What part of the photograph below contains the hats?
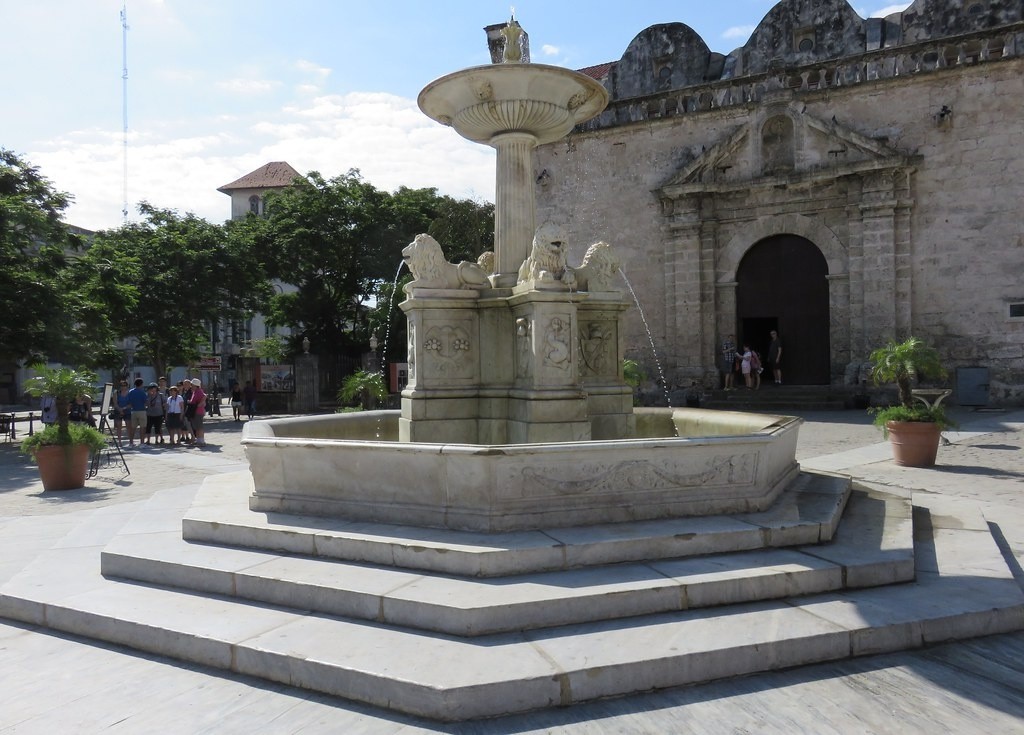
[190,378,201,387]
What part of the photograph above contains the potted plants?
[18,362,110,489]
[863,336,950,467]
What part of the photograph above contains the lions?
[516,223,575,284]
[402,233,493,294]
[574,241,619,292]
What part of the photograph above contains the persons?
[181,378,195,446]
[165,385,184,447]
[767,330,784,386]
[721,335,738,391]
[128,378,152,449]
[39,387,60,429]
[143,382,168,448]
[738,343,753,392]
[68,390,97,431]
[175,381,190,442]
[155,376,175,447]
[749,349,762,390]
[228,382,244,422]
[112,380,135,450]
[240,381,257,421]
[186,377,207,447]
